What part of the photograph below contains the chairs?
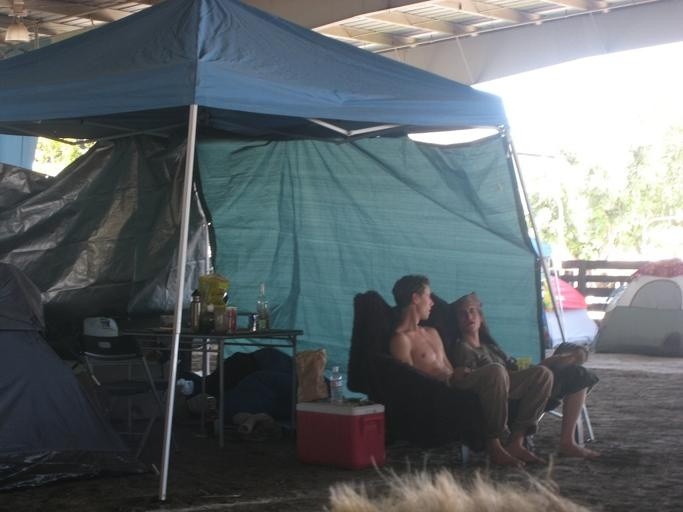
[51,307,175,465]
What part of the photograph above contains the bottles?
[255,282,268,332]
[189,295,201,328]
[463,356,477,376]
[329,366,342,404]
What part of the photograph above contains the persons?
[389,276,554,466]
[451,293,603,460]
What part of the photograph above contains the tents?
[0,262,150,492]
[593,259,683,357]
[540,276,599,349]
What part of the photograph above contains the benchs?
[334,282,599,483]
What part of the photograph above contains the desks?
[110,325,306,467]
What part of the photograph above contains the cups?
[159,313,174,329]
[516,357,532,369]
[248,314,257,332]
[199,312,215,334]
[224,307,239,332]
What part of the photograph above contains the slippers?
[481,452,528,471]
[516,451,548,465]
[559,445,601,460]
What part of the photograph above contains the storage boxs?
[291,397,389,473]
